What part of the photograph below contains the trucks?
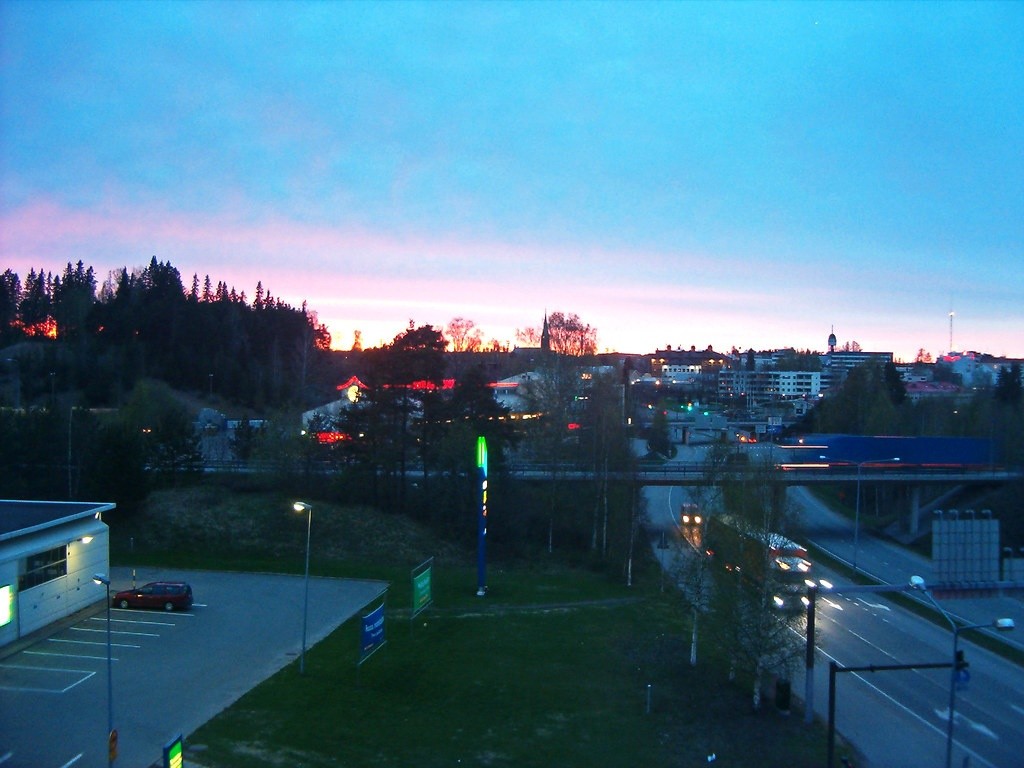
[706,511,809,614]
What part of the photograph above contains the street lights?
[820,454,901,576]
[294,501,311,676]
[910,575,1015,768]
[93,573,115,768]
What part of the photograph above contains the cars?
[682,503,701,525]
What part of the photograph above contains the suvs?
[112,581,193,612]
